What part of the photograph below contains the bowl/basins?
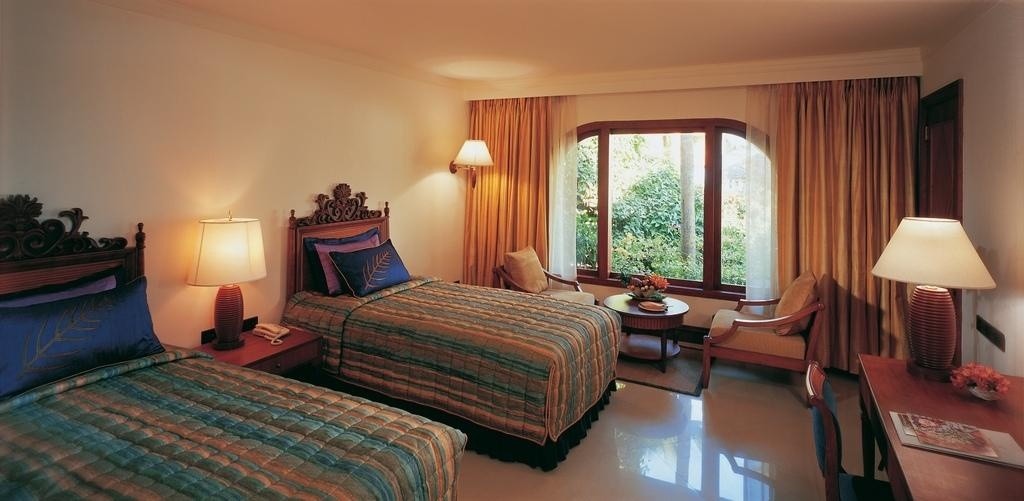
[626,292,667,301]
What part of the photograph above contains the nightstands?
[191,323,324,387]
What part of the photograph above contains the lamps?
[449,139,495,189]
[871,216,996,381]
[185,209,268,352]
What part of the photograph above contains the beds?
[279,184,623,472]
[0,193,468,501]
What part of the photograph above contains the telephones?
[253,323,290,340]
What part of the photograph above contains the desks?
[603,293,690,374]
[856,353,1024,501]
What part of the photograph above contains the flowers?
[948,362,1010,401]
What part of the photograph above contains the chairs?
[804,359,897,501]
[492,263,600,307]
[702,296,824,409]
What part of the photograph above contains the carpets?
[615,345,716,397]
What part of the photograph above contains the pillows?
[774,268,819,336]
[503,245,549,295]
[303,227,413,299]
[0,264,165,400]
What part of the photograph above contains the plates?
[638,301,668,312]
